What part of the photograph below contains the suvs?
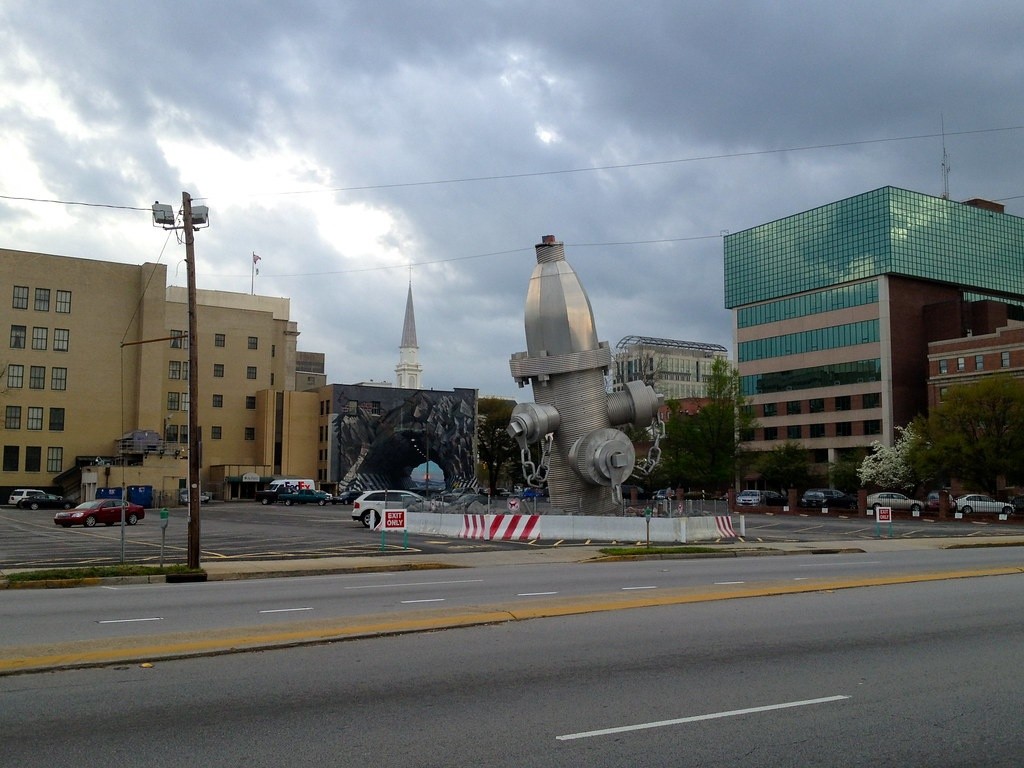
[351,490,451,531]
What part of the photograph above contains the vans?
[802,489,848,508]
[264,479,316,505]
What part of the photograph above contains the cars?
[8,488,48,506]
[759,491,784,505]
[428,486,549,502]
[176,487,189,506]
[17,494,78,510]
[332,491,363,505]
[621,485,644,498]
[651,489,675,500]
[953,493,1014,515]
[313,490,333,501]
[737,489,762,507]
[51,498,145,527]
[861,492,925,511]
[926,490,956,511]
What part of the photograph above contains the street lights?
[146,188,211,586]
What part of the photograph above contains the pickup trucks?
[254,487,299,506]
[277,488,330,507]
